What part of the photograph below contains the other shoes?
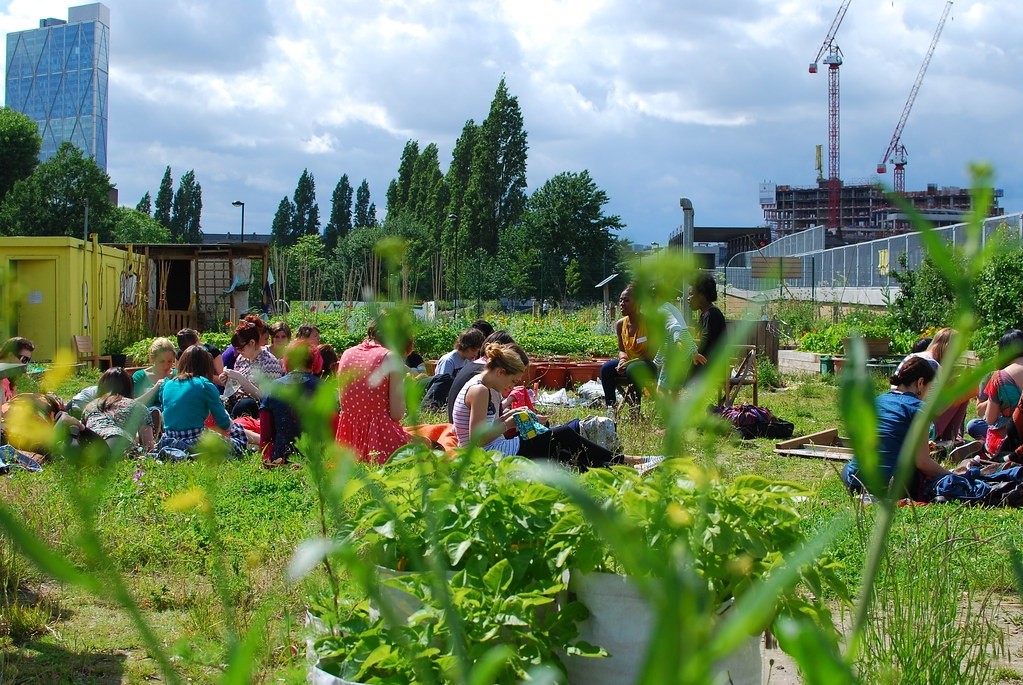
[638,455,673,478]
[607,406,614,417]
[139,409,160,445]
[949,441,982,465]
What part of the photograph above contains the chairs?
[615,344,758,424]
[73,335,113,371]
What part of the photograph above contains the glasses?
[235,342,248,351]
[14,353,31,364]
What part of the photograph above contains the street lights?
[447,213,459,320]
[231,200,245,242]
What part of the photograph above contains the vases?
[519,355,613,388]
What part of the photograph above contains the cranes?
[876,0,953,198]
[808,0,852,233]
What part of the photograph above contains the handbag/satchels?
[513,411,548,441]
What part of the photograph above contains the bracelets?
[502,422,508,431]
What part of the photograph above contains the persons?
[453,343,665,472]
[842,328,1023,505]
[66,312,445,466]
[0,337,36,476]
[434,320,542,423]
[600,273,728,425]
